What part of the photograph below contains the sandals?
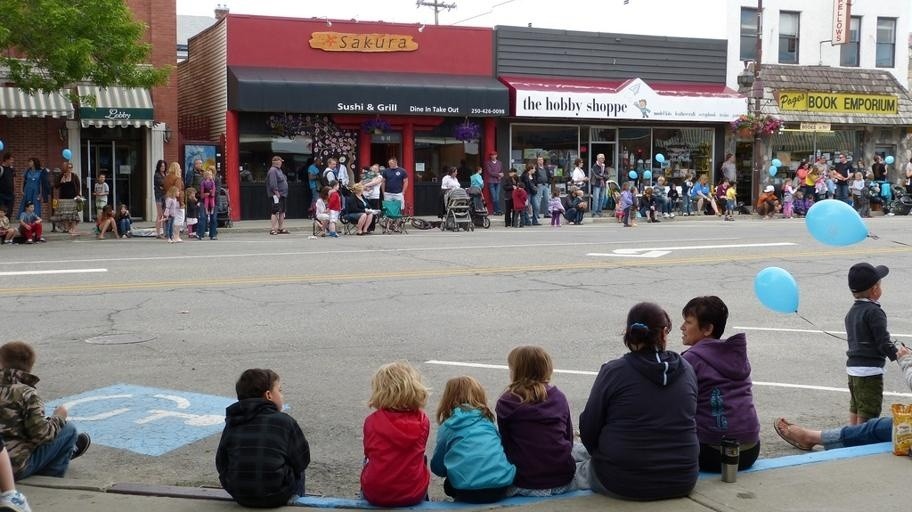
[277,229,290,233]
[270,229,277,235]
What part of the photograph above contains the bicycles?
[378,206,431,229]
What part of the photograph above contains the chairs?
[341,196,359,236]
[380,200,409,235]
[311,206,330,237]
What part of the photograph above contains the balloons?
[0,140,4,152]
[655,153,665,163]
[643,169,653,181]
[771,158,783,168]
[805,199,868,247]
[769,166,778,178]
[885,155,896,166]
[629,170,638,180]
[62,148,72,161]
[754,266,800,315]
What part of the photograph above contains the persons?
[572,158,589,193]
[215,368,311,510]
[18,201,47,244]
[315,187,332,235]
[0,342,90,483]
[589,154,608,218]
[93,173,109,218]
[640,188,661,222]
[185,160,204,232]
[360,167,380,197]
[667,183,683,216]
[185,188,200,238]
[757,185,779,219]
[805,193,815,211]
[782,178,798,219]
[846,262,891,425]
[421,167,435,182]
[154,160,168,239]
[521,163,542,226]
[878,155,885,164]
[197,166,218,240]
[831,153,854,204]
[0,152,15,221]
[1,435,33,512]
[0,205,19,245]
[652,176,675,218]
[164,186,180,243]
[772,343,912,453]
[162,162,184,243]
[869,183,884,202]
[382,156,409,214]
[905,155,912,194]
[629,186,640,227]
[690,174,721,217]
[495,347,577,495]
[96,205,120,240]
[266,156,291,236]
[726,180,737,221]
[239,163,257,184]
[574,191,587,226]
[359,164,383,232]
[682,173,695,217]
[848,171,864,209]
[615,196,624,223]
[202,170,216,215]
[512,183,529,229]
[535,155,554,219]
[323,180,342,237]
[457,159,472,177]
[503,167,526,228]
[549,187,566,227]
[859,180,875,218]
[620,182,634,227]
[335,158,349,189]
[872,156,889,181]
[721,153,736,183]
[679,296,761,472]
[360,362,429,507]
[486,152,505,216]
[430,377,516,503]
[814,155,828,175]
[307,157,322,203]
[803,165,824,205]
[114,204,134,239]
[437,166,461,219]
[346,183,373,236]
[578,303,698,501]
[851,159,868,178]
[865,173,895,216]
[322,158,337,190]
[562,185,587,225]
[794,162,809,197]
[815,166,828,195]
[470,167,487,210]
[717,177,732,215]
[16,157,48,220]
[55,163,81,234]
[824,171,839,200]
[793,191,806,215]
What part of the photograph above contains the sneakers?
[0,490,29,512]
[71,433,91,459]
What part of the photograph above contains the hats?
[490,151,498,155]
[764,185,775,192]
[272,156,284,163]
[849,262,890,292]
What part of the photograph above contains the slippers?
[774,417,811,450]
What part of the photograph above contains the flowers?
[760,118,783,134]
[360,119,392,133]
[730,116,762,133]
[452,123,480,143]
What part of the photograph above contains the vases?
[738,128,754,137]
[372,128,384,135]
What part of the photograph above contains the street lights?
[737,59,761,213]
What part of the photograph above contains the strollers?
[466,187,490,228]
[216,187,231,228]
[439,189,474,232]
